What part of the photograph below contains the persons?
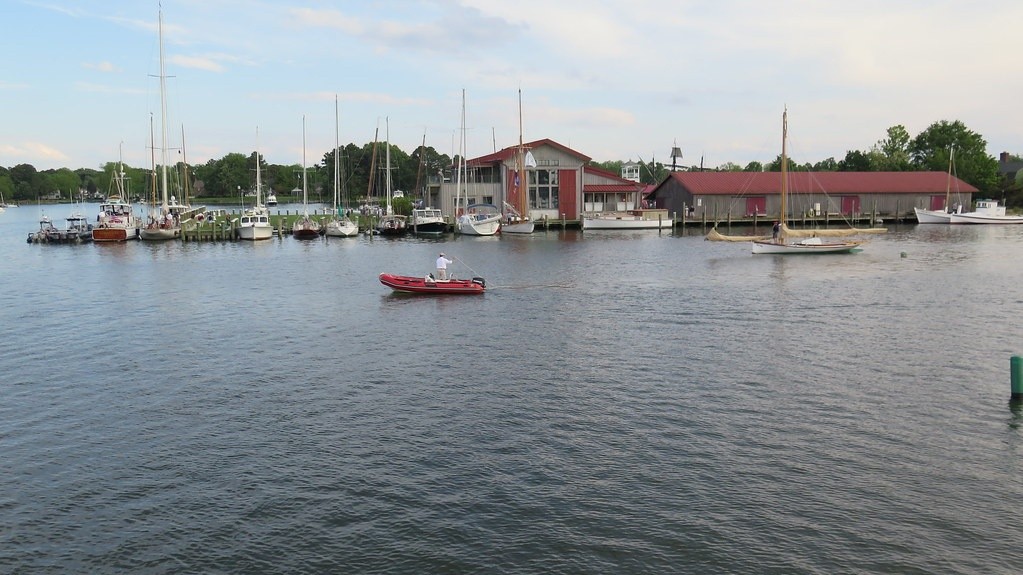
[191,212,195,221]
[117,207,123,214]
[166,212,172,219]
[773,222,779,241]
[685,205,689,219]
[689,204,695,219]
[346,210,350,217]
[436,253,452,280]
[226,213,231,225]
[207,213,212,228]
[198,211,204,228]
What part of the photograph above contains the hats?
[440,253,445,256]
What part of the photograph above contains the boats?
[379,272,488,295]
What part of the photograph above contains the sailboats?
[914,143,1023,225]
[27,1,273,242]
[291,113,325,238]
[703,101,867,254]
[0,191,20,209]
[267,187,278,206]
[361,86,535,237]
[326,94,360,237]
